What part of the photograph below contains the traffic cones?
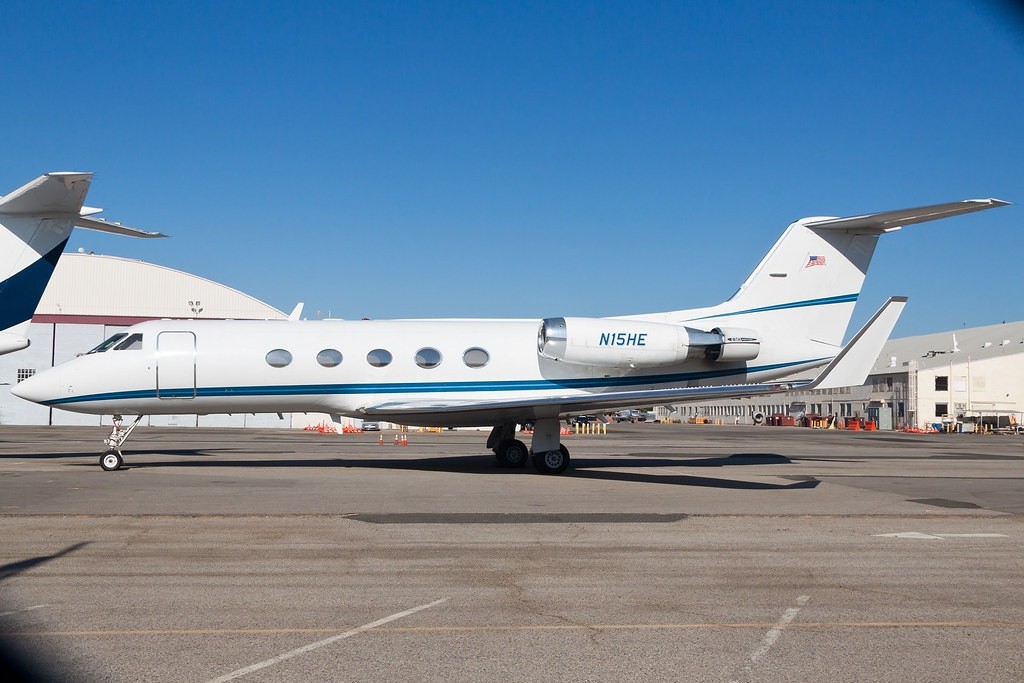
[401,434,405,447]
[379,432,383,446]
[393,435,399,445]
[404,435,408,447]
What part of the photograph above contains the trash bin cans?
[932,424,942,430]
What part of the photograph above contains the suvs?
[614,410,649,423]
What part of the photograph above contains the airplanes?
[0,172,1014,476]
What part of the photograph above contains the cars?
[571,416,590,427]
[361,421,380,430]
